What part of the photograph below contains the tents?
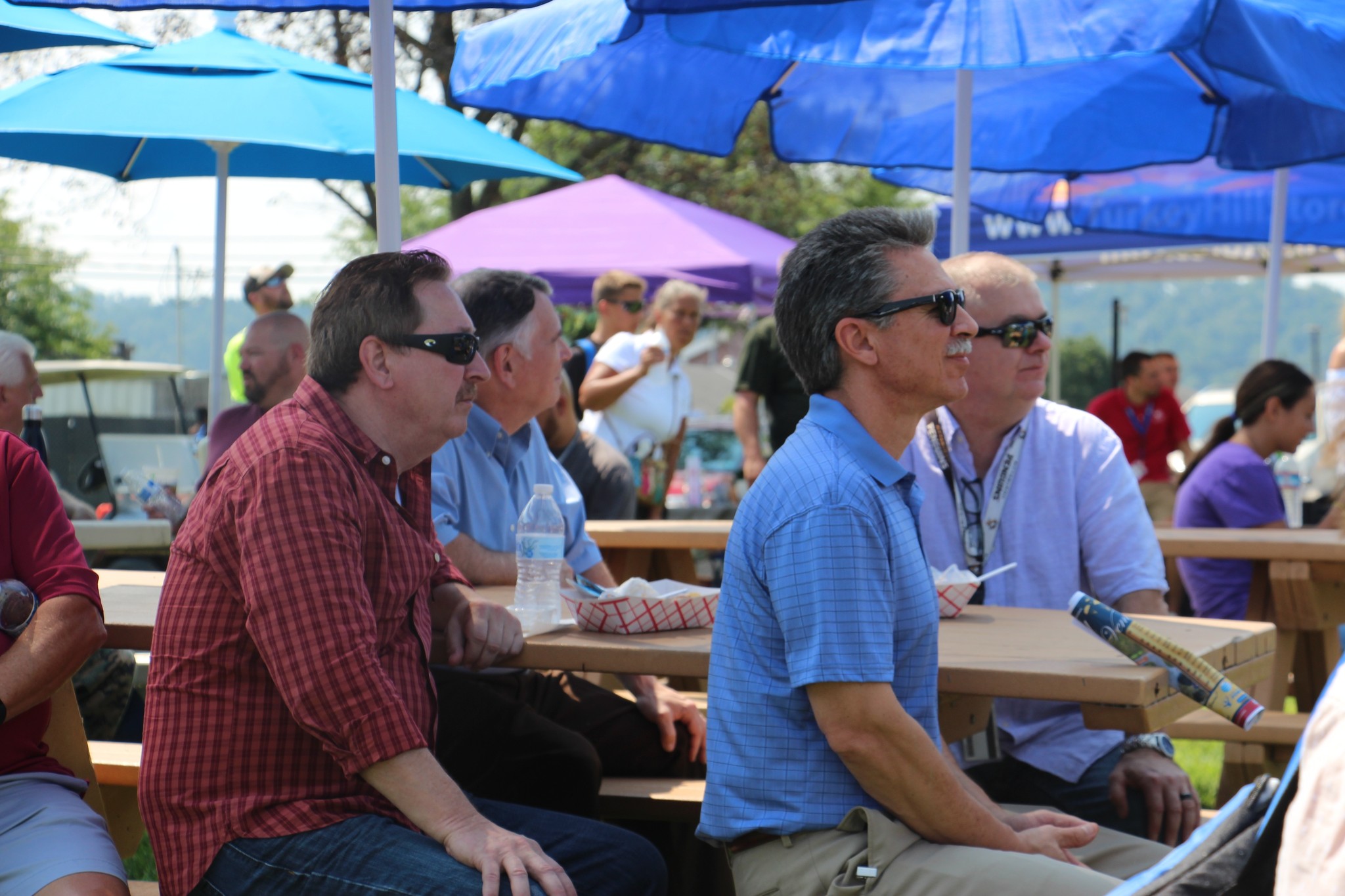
[401,171,806,308]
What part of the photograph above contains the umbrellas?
[0,0,1345,460]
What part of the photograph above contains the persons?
[0,249,1345,895]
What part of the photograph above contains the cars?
[1169,388,1237,472]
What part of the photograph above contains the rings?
[1180,793,1193,800]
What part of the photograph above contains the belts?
[724,828,796,856]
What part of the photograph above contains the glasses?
[865,289,965,326]
[607,300,642,313]
[390,332,480,365]
[262,275,284,288]
[961,477,984,559]
[973,314,1054,349]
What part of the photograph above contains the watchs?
[1122,731,1175,759]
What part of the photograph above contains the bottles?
[1273,451,1303,529]
[513,484,565,632]
[121,468,188,525]
[114,475,130,514]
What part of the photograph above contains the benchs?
[86,691,1309,803]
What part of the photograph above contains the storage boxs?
[561,578,720,634]
[936,570,980,618]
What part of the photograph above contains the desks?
[91,616,1345,810]
[491,520,1345,711]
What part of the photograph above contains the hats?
[244,264,293,297]
